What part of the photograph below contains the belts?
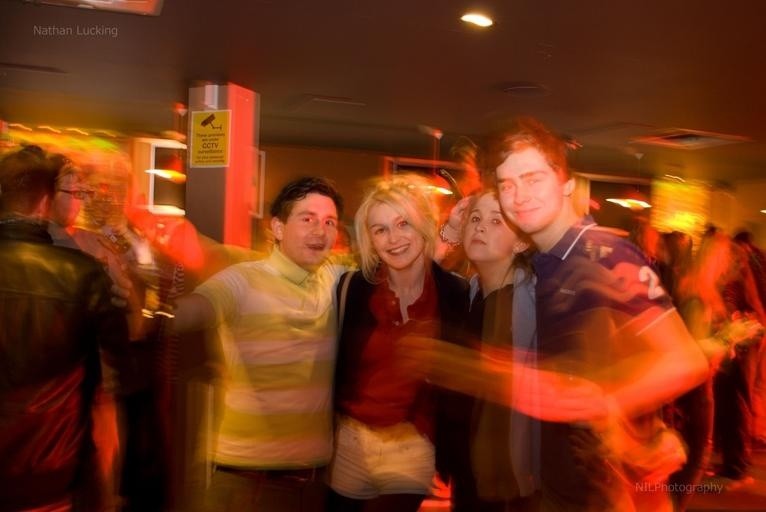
[213,463,328,484]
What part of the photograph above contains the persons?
[0,115,766,511]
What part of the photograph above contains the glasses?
[55,185,96,201]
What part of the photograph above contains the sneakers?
[696,460,753,493]
